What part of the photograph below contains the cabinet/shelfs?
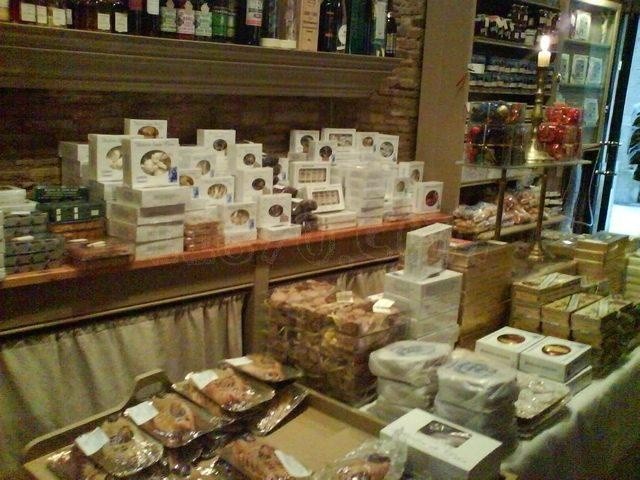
[415,0,567,242]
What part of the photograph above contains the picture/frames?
[560,9,603,124]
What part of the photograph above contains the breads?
[262,280,406,407]
[454,190,548,236]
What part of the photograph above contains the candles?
[537,34,552,67]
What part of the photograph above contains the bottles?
[384,8,397,57]
[475,4,558,51]
[470,54,555,89]
[1,0,298,46]
[319,0,339,53]
[335,0,347,52]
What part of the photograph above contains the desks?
[500,347,639,479]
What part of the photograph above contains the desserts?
[45,353,386,480]
